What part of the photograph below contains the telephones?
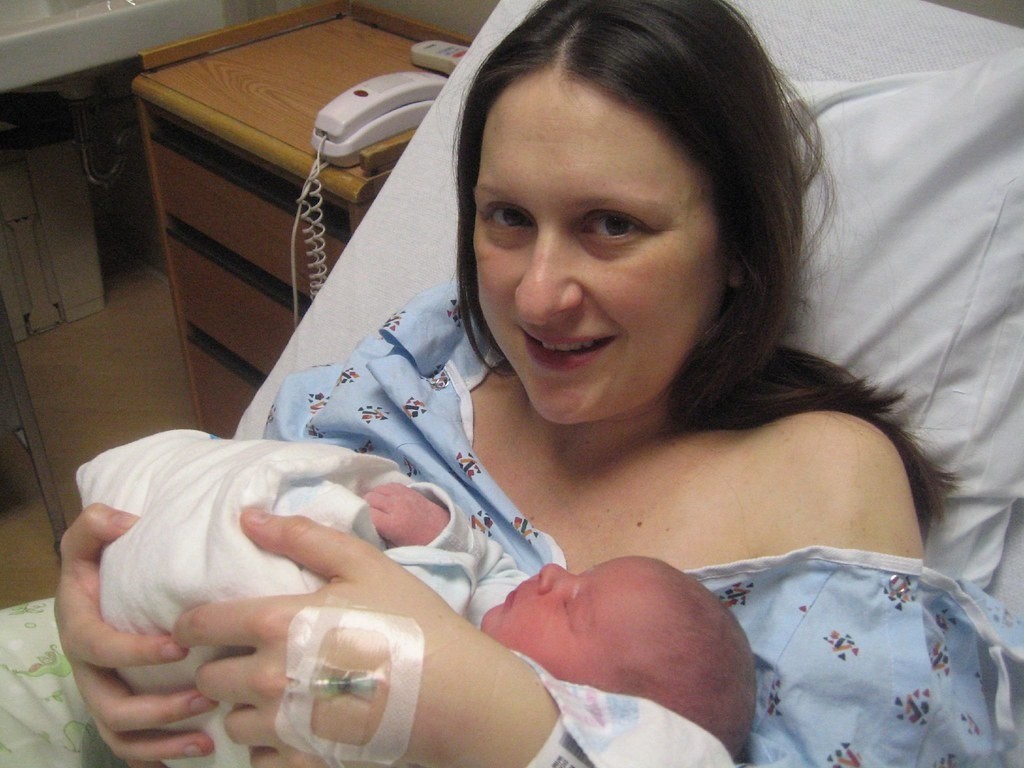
[307,63,452,170]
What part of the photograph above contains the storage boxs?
[0,91,106,345]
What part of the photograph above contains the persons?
[55,1,1023,767]
[76,428,757,768]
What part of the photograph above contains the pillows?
[779,43,1024,504]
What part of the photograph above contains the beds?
[0,0,1024,768]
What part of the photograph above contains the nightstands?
[135,0,478,440]
